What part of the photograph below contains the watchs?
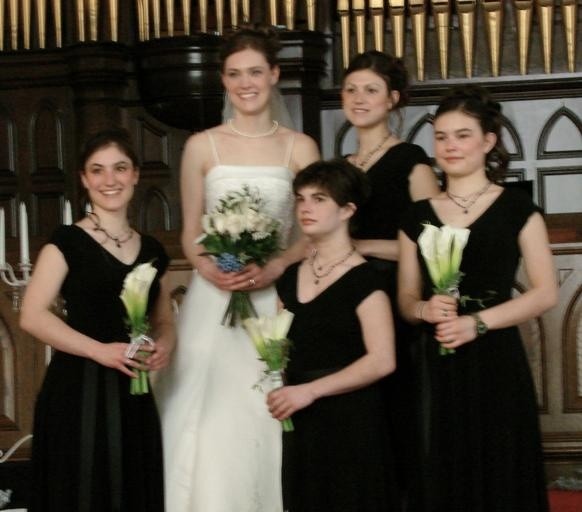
[469,312,488,336]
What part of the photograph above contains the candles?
[18,201,32,264]
[83,200,92,219]
[1,207,6,268]
[62,199,73,228]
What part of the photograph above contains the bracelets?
[416,301,425,320]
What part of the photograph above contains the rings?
[249,279,255,287]
[441,309,448,316]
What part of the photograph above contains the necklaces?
[82,211,134,248]
[449,191,477,203]
[311,246,355,285]
[314,257,340,271]
[446,181,495,215]
[225,115,281,140]
[352,134,394,170]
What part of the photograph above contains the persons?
[146,30,321,511]
[261,159,410,511]
[17,124,177,511]
[326,48,441,509]
[397,84,563,512]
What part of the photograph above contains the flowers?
[193,182,288,327]
[118,256,160,396]
[414,219,501,357]
[239,305,299,432]
